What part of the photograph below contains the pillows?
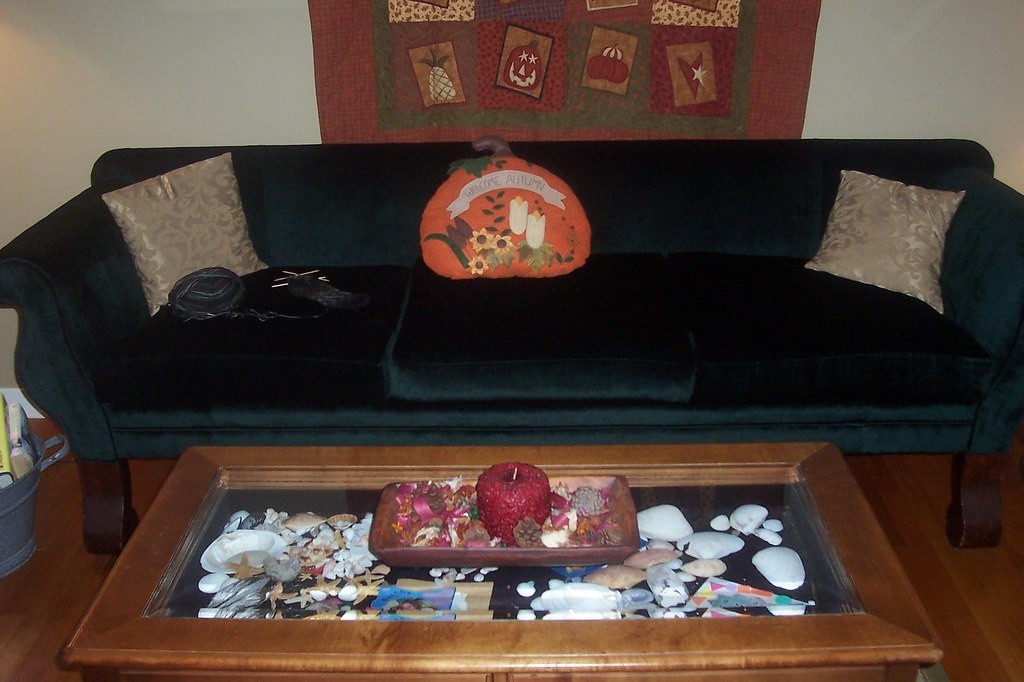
[806,168,967,315]
[412,138,591,279]
[100,150,268,317]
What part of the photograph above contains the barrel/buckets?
[0,430,48,580]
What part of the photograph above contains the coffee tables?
[56,441,945,682]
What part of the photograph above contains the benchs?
[0,139,1024,550]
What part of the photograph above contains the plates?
[368,473,641,568]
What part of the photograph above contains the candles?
[474,462,552,544]
